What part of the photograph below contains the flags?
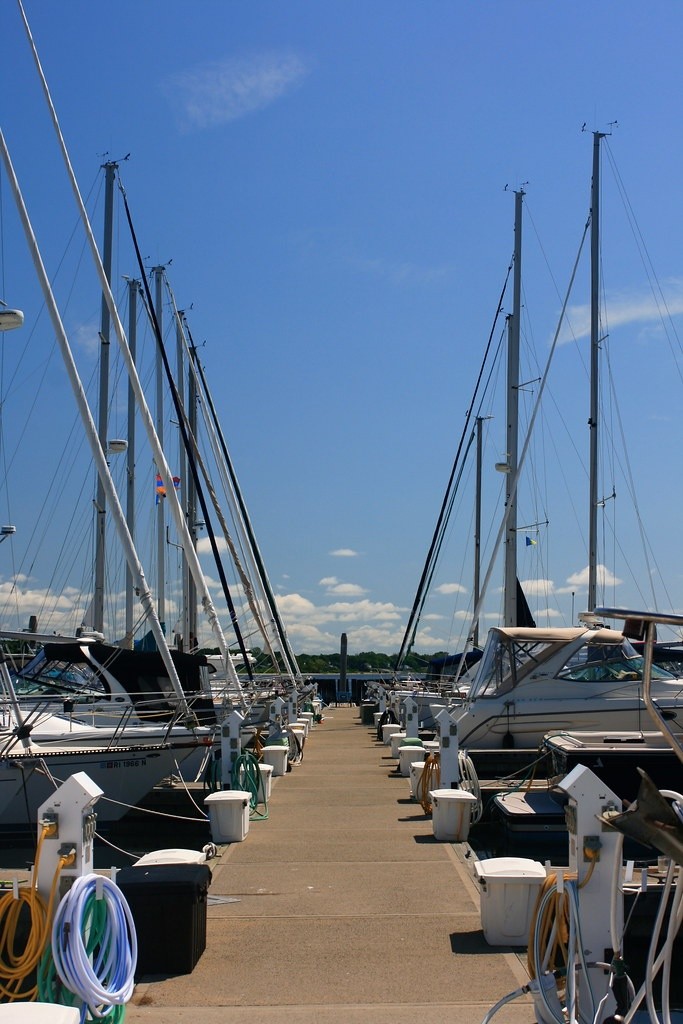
[526,536,537,547]
[155,475,179,506]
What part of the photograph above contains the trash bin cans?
[398,746,426,777]
[374,712,385,728]
[474,856,546,946]
[282,730,304,749]
[260,745,289,775]
[299,712,314,727]
[204,790,251,843]
[410,762,437,800]
[285,723,308,738]
[297,718,310,729]
[382,723,402,745]
[429,788,476,842]
[239,764,274,803]
[390,733,407,758]
[134,849,208,866]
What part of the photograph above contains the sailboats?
[1,0,321,853]
[359,113,683,853]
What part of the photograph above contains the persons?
[178,632,198,654]
[407,673,415,687]
[391,671,399,687]
